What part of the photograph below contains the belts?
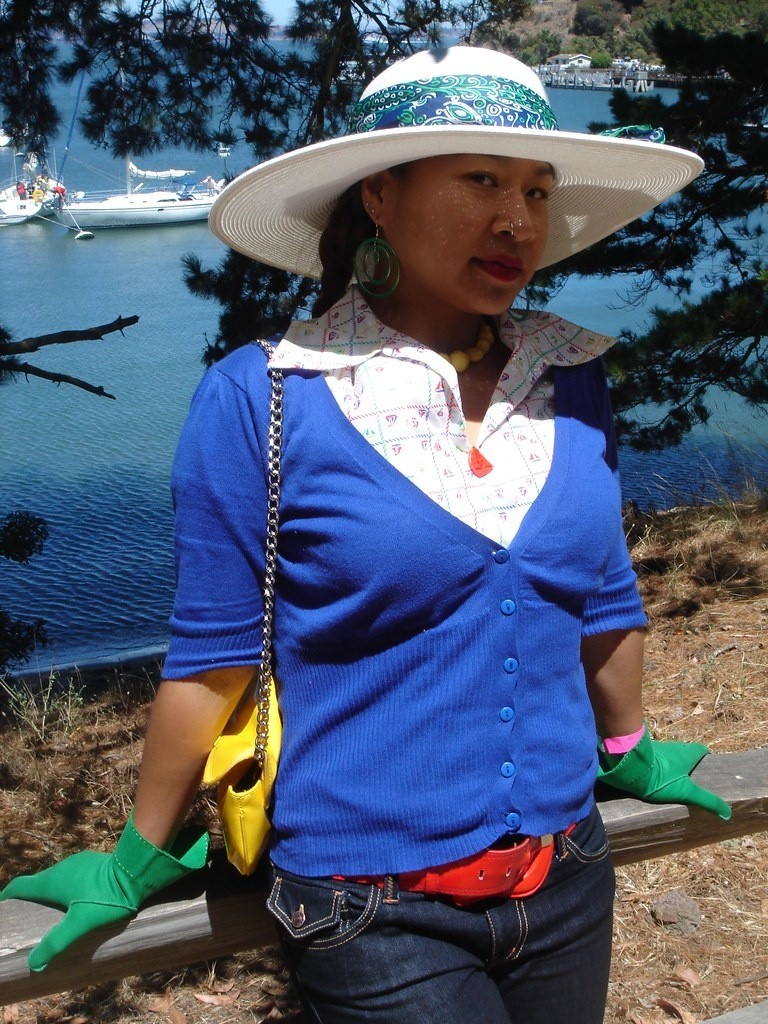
[330,824,576,906]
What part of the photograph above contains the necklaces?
[436,324,494,373]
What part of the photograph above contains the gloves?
[597,719,732,820]
[0,807,210,973]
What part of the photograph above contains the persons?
[199,176,217,189]
[0,44,732,1024]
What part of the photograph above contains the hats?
[209,47,704,281]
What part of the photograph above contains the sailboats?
[49,49,225,228]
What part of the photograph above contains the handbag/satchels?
[197,338,284,876]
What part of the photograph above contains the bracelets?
[596,725,645,754]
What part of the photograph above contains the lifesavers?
[33,189,44,201]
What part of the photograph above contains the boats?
[0,126,65,225]
[0,128,44,147]
